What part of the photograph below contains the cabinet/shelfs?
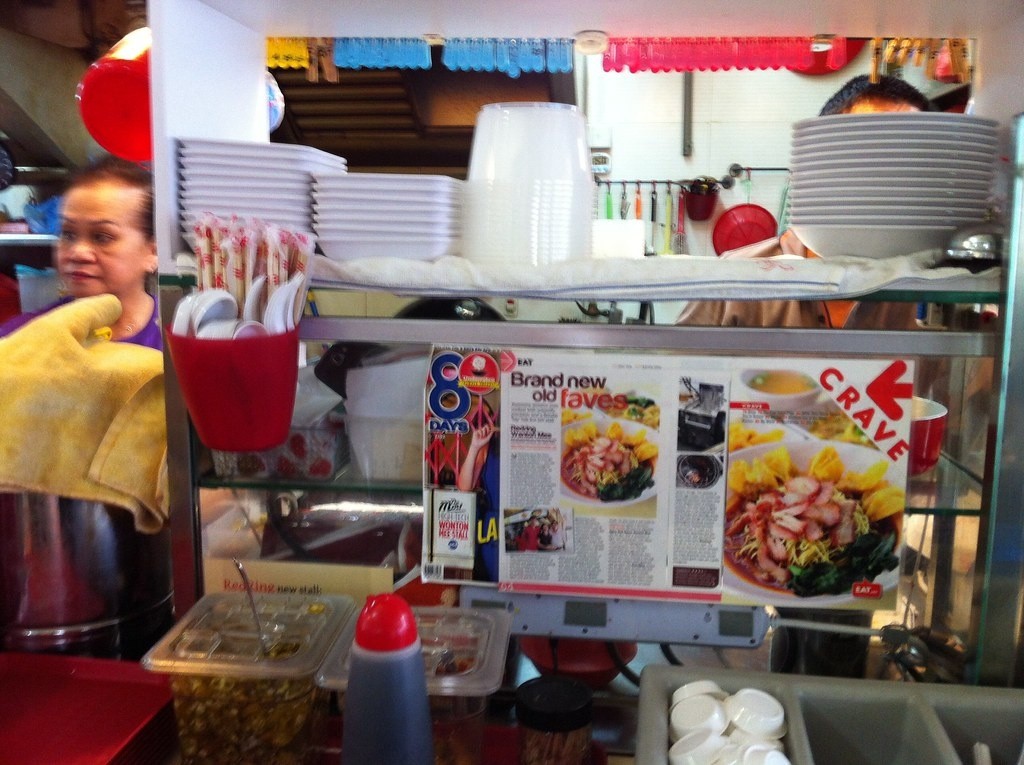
[148,0,1024,716]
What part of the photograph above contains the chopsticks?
[194,213,316,326]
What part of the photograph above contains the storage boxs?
[330,411,423,483]
[211,419,348,484]
[312,605,514,765]
[139,592,356,765]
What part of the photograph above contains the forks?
[244,270,305,334]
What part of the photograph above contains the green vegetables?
[787,519,899,597]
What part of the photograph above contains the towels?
[1,293,170,533]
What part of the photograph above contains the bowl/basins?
[668,679,793,765]
[467,100,596,262]
[786,113,1001,259]
[560,419,659,509]
[171,288,270,339]
[740,368,821,410]
[907,392,949,477]
[725,440,907,611]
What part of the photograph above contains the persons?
[0,155,167,355]
[672,72,997,495]
[457,409,564,583]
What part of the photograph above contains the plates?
[175,135,465,259]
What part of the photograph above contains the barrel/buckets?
[164,322,301,452]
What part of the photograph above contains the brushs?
[670,191,689,254]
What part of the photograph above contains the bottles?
[342,594,435,765]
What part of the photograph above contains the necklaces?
[821,299,834,328]
